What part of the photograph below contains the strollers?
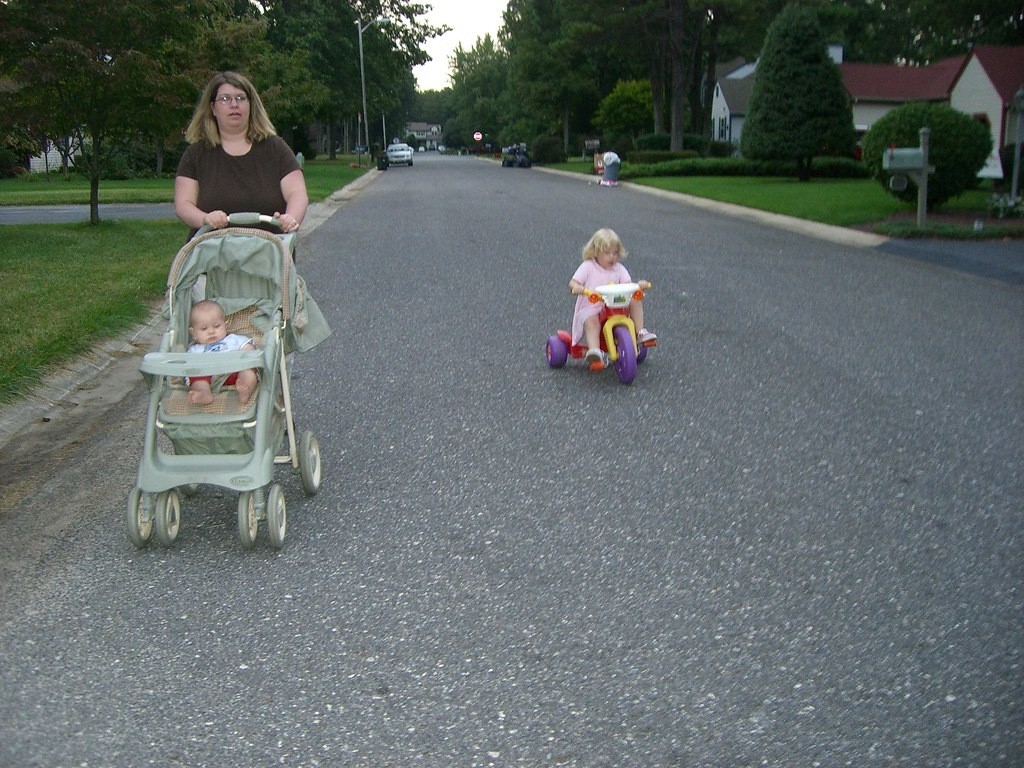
[125,211,323,548]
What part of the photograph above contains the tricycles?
[544,280,658,385]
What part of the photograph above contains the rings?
[293,220,297,224]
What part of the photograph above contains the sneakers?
[636,329,658,346]
[583,349,609,369]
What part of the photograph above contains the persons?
[174,71,310,436]
[568,229,656,361]
[174,300,258,405]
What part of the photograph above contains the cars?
[386,143,413,167]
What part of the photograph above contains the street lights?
[357,17,390,167]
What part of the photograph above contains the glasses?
[212,95,251,105]
